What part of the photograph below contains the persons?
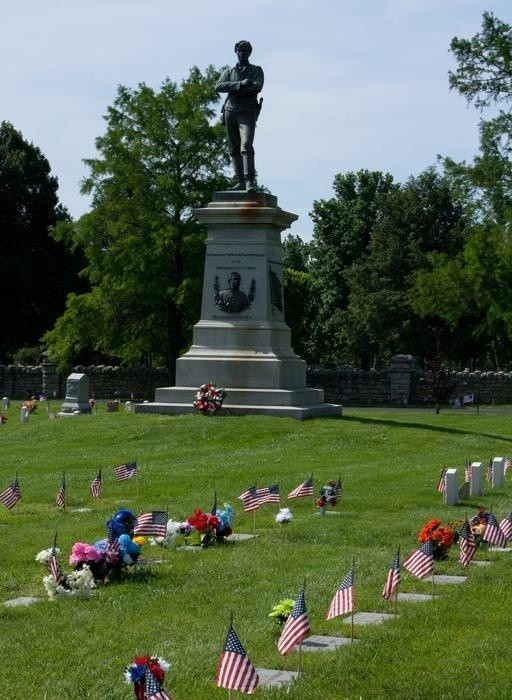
[215,40,264,191]
[217,271,249,312]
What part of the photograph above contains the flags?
[483,514,506,549]
[287,476,314,498]
[334,480,343,498]
[403,537,435,579]
[468,466,471,479]
[499,512,511,541]
[145,665,169,700]
[256,484,280,505]
[459,521,478,567]
[462,392,474,403]
[327,566,354,621]
[277,588,310,656]
[48,551,62,584]
[113,460,138,483]
[504,460,511,476]
[487,462,492,481]
[0,475,21,510]
[217,625,259,694]
[382,553,402,602]
[238,487,260,512]
[108,539,121,557]
[56,476,66,508]
[90,468,101,498]
[132,510,169,537]
[438,469,445,493]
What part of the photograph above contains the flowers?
[0,415,7,423]
[17,400,37,412]
[88,398,95,409]
[186,504,236,533]
[469,503,490,536]
[122,654,171,700]
[69,536,145,567]
[192,383,226,415]
[107,402,120,412]
[418,519,455,548]
